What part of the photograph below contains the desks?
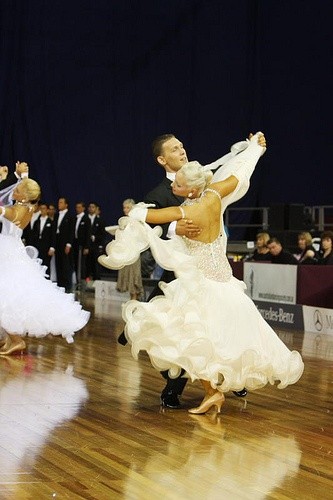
[229,261,333,329]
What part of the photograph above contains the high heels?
[188,392,225,413]
[0,341,26,355]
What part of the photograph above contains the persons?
[124,130,270,417]
[0,160,41,357]
[246,228,333,264]
[24,197,106,294]
[113,196,146,303]
[116,133,267,412]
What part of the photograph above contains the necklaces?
[180,192,205,208]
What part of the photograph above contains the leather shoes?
[161,387,182,409]
[233,387,247,397]
